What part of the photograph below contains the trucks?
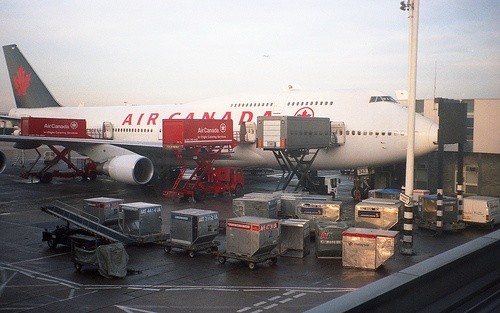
[463,196,500,230]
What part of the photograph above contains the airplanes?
[0,44,440,201]
